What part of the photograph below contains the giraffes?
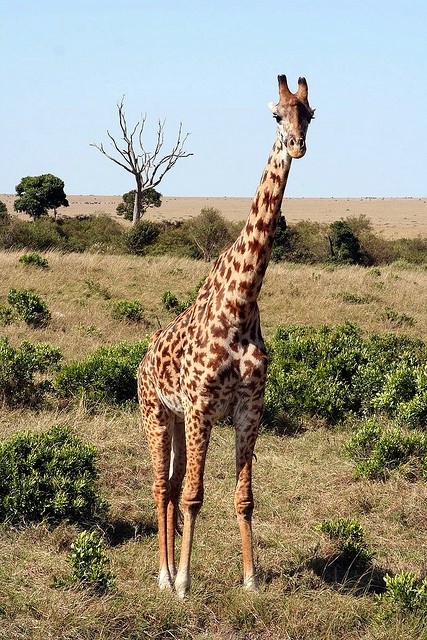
[137,75,316,599]
